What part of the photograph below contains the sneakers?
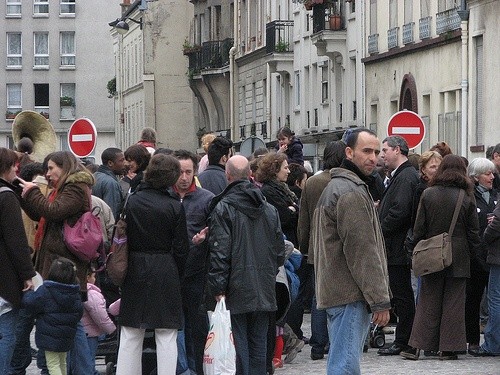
[284,339,304,364]
[30,347,38,359]
[311,346,325,360]
[468,347,500,356]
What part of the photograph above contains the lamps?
[116,16,143,35]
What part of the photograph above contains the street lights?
[116,17,142,34]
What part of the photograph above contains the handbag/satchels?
[203,295,237,375]
[106,191,134,286]
[412,232,452,278]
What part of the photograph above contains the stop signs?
[386,110,426,150]
[68,118,97,158]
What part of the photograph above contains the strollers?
[95,313,119,375]
[364,323,386,352]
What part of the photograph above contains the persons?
[463,158,500,356]
[374,136,420,356]
[474,196,500,358]
[312,129,394,375]
[296,129,500,360]
[166,148,216,375]
[14,124,311,369]
[13,150,94,375]
[0,147,36,375]
[107,153,192,375]
[400,154,482,361]
[206,154,286,375]
[22,256,84,375]
[79,262,117,375]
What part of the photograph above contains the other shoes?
[440,352,457,360]
[400,348,420,360]
[274,359,283,368]
[480,319,487,334]
[302,337,309,344]
[425,350,440,356]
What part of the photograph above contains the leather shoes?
[377,343,411,355]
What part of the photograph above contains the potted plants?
[6,110,22,119]
[60,96,75,118]
[329,4,341,29]
[40,112,49,119]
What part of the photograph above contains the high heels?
[266,359,274,375]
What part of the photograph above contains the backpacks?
[61,183,107,273]
